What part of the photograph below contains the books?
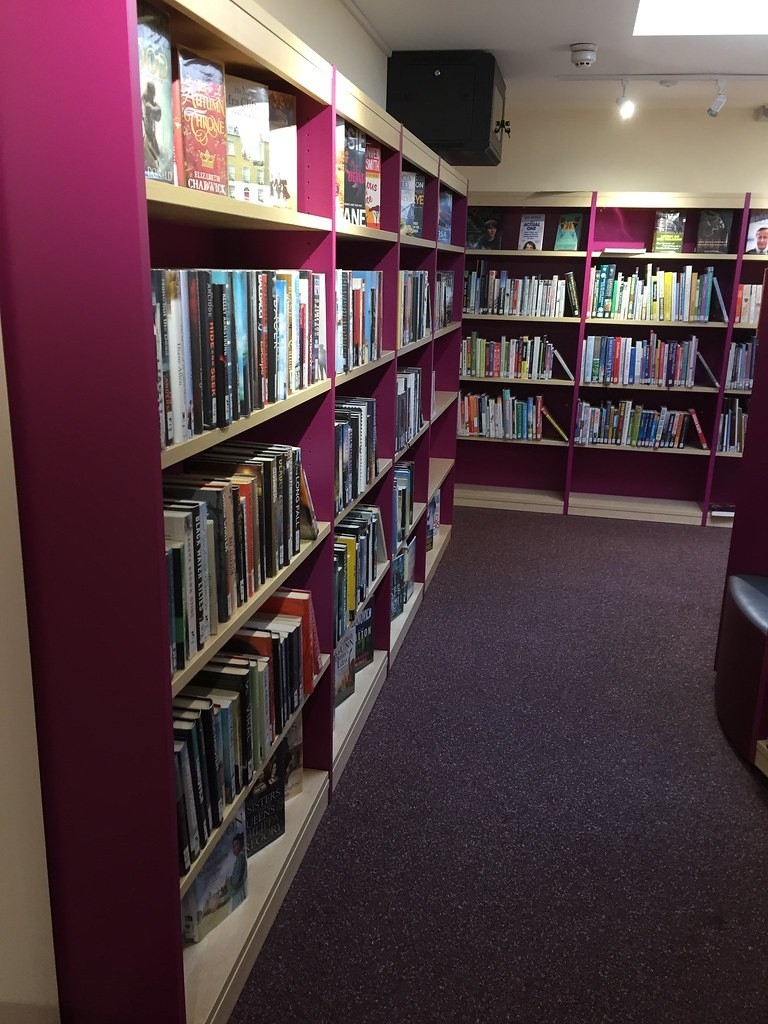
[138,1,768,947]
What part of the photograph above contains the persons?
[522,241,536,250]
[268,762,279,785]
[140,81,162,173]
[745,227,768,255]
[474,220,500,249]
[219,833,247,916]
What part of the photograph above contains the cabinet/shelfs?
[0,0,768,1024]
